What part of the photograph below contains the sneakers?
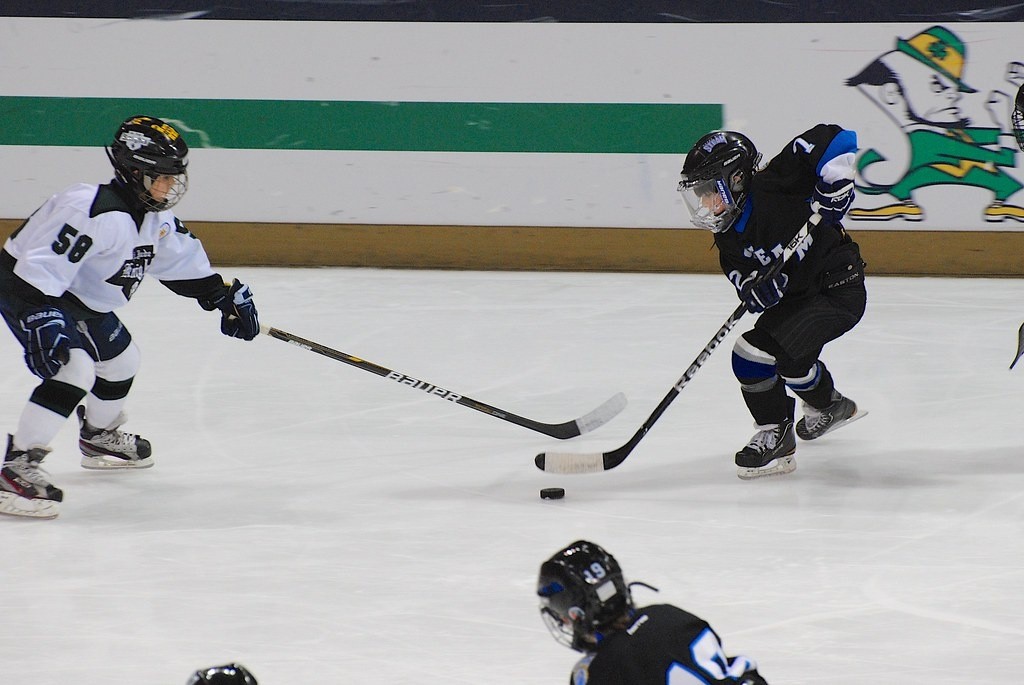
[735,395,798,480]
[75,404,155,471]
[0,433,63,519]
[796,388,869,440]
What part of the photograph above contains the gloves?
[746,271,788,314]
[810,174,856,225]
[211,276,260,341]
[18,303,70,379]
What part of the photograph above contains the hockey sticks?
[225,313,629,439]
[532,211,821,475]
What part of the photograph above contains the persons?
[186,662,258,685]
[677,123,869,481]
[0,116,260,519]
[535,540,768,685]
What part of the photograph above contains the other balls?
[540,488,565,499]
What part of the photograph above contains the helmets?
[187,661,256,685]
[1011,81,1024,151]
[677,129,764,206]
[536,539,628,652]
[111,114,190,213]
